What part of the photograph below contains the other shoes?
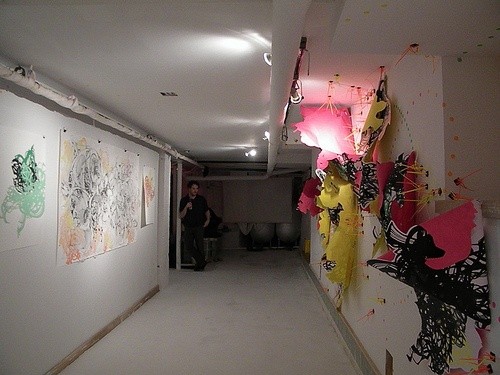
[194,260,208,271]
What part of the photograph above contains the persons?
[178,180,210,272]
